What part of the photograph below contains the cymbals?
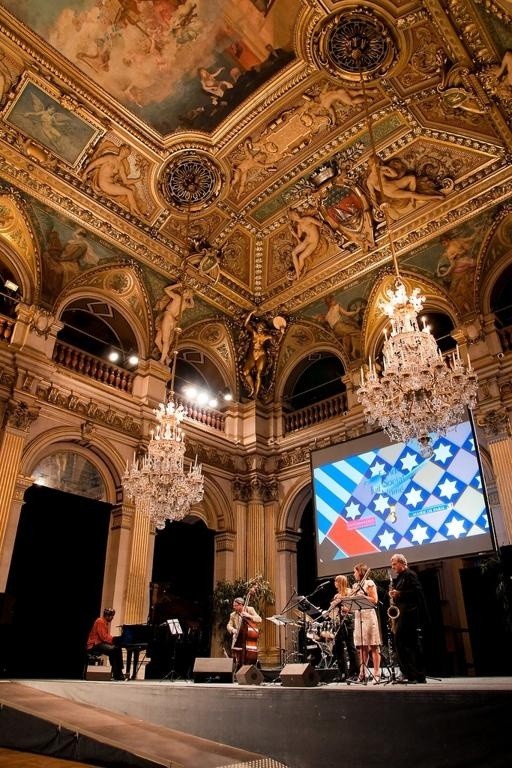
[292,608,313,623]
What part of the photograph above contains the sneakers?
[333,674,357,682]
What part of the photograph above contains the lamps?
[120,184,205,530]
[357,50,478,458]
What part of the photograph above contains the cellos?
[231,581,263,674]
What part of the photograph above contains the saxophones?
[387,575,401,634]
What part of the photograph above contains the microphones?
[315,579,331,589]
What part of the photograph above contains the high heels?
[356,673,381,685]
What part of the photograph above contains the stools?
[81,651,104,679]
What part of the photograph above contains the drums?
[306,622,323,641]
[319,620,340,639]
[303,637,333,668]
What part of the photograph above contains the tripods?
[346,610,412,687]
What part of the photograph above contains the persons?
[227,596,262,673]
[386,553,427,684]
[349,563,382,684]
[82,144,149,221]
[154,283,193,364]
[244,310,284,398]
[366,154,446,214]
[288,210,334,282]
[230,141,277,202]
[322,574,361,682]
[86,608,130,682]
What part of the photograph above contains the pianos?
[111,624,197,683]
